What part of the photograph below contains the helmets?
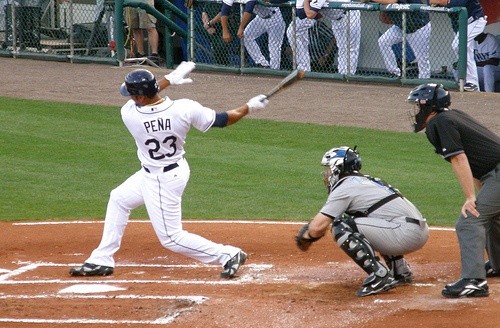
[405,83,450,133]
[120,69,160,97]
[320,145,362,188]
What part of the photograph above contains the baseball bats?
[259,69,304,102]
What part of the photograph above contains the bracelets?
[324,53,329,57]
[308,232,321,240]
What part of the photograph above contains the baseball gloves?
[295,222,312,250]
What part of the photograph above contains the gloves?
[164,61,196,85]
[246,95,270,114]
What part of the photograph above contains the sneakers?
[130,52,146,65]
[358,273,399,296]
[485,261,499,277]
[453,62,458,70]
[394,273,412,283]
[221,251,248,278]
[69,263,113,276]
[464,83,477,92]
[149,53,159,66]
[442,279,489,298]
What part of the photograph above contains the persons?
[201,0,240,66]
[125,0,160,67]
[360,0,434,80]
[220,0,286,70]
[296,145,431,296]
[427,0,487,93]
[69,59,269,280]
[473,31,500,92]
[303,0,363,76]
[286,0,336,72]
[407,83,500,298]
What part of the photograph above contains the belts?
[406,217,420,226]
[337,11,346,20]
[262,10,276,19]
[143,163,178,173]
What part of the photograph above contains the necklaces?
[145,98,165,106]
